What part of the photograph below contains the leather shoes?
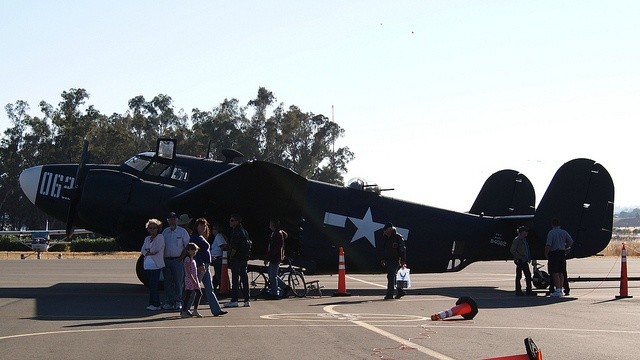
[214,309,228,316]
[180,310,193,316]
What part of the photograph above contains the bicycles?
[238,257,307,298]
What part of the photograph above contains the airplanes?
[20,138,614,291]
[0,220,93,260]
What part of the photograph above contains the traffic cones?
[217,251,231,298]
[431,297,477,320]
[331,248,351,297]
[474,339,542,360]
[615,244,634,299]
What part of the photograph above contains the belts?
[164,257,181,259]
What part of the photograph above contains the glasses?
[148,227,157,229]
[213,228,216,230]
[230,219,237,222]
[525,231,528,232]
[188,248,195,250]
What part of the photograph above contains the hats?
[382,222,393,229]
[178,214,193,225]
[166,212,179,218]
[518,226,529,232]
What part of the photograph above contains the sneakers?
[526,290,537,296]
[515,291,524,296]
[146,304,153,309]
[546,290,554,296]
[384,296,393,299]
[560,292,563,295]
[150,305,161,311]
[162,304,174,310]
[175,302,182,309]
[192,311,202,318]
[550,292,562,299]
[395,291,407,299]
[223,300,238,308]
[565,292,569,295]
[243,301,250,307]
[181,311,193,318]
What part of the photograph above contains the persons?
[377,222,411,299]
[178,212,193,235]
[545,218,574,297]
[181,218,228,316]
[176,243,206,318]
[510,225,537,296]
[210,224,226,289]
[264,217,290,300]
[544,227,577,296]
[140,218,166,311]
[162,211,190,310]
[224,213,253,308]
[211,224,228,287]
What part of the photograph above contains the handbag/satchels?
[396,266,411,288]
